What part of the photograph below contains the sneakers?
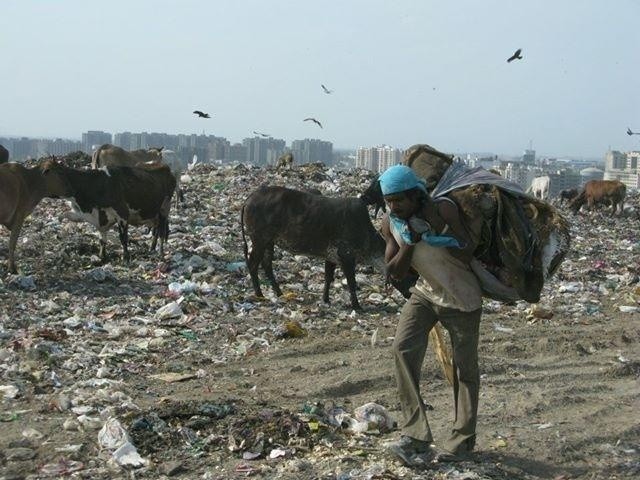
[437,450,472,462]
[397,436,427,453]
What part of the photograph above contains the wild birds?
[320,84,330,95]
[507,48,523,63]
[253,130,271,137]
[194,110,211,119]
[303,116,323,129]
[626,126,640,137]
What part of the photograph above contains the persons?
[379,165,482,462]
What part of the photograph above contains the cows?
[0,143,178,275]
[524,175,551,201]
[275,152,293,172]
[359,175,386,220]
[240,186,386,315]
[560,180,626,218]
